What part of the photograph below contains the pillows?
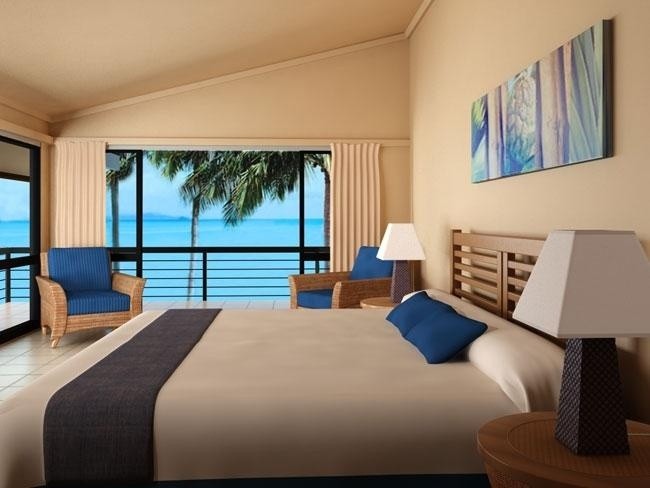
[385,290,451,339]
[404,303,488,364]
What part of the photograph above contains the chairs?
[35,246,147,348]
[288,247,417,309]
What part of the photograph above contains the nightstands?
[358,296,399,309]
[476,412,649,488]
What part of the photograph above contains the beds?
[0,229,565,488]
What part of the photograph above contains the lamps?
[377,223,427,303]
[513,228,650,457]
